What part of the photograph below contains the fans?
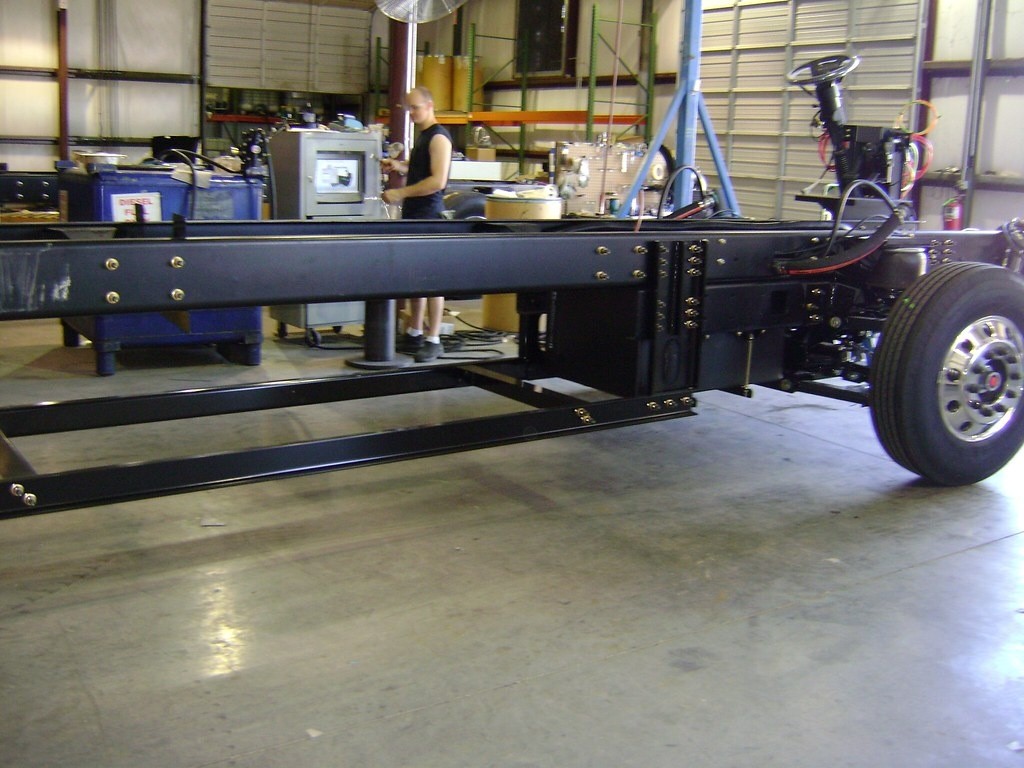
[375,0,468,24]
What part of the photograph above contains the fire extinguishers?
[942,194,968,232]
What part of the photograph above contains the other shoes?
[395,332,424,355]
[414,338,445,362]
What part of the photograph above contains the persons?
[378,88,454,364]
